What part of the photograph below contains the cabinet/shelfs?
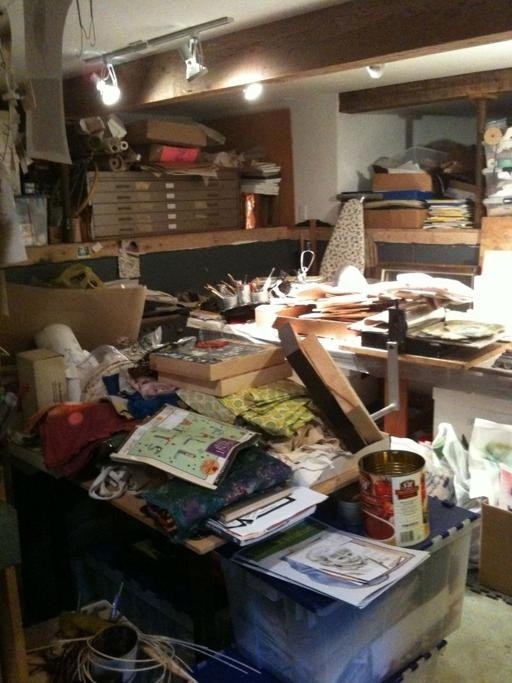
[299,67,512,284]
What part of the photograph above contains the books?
[424,196,472,230]
[239,160,282,199]
[204,506,320,549]
[215,483,293,523]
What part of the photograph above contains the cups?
[357,451,432,550]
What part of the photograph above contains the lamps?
[178,26,208,81]
[90,57,121,107]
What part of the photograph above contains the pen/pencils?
[203,283,224,300]
[243,274,248,284]
[248,277,261,292]
[227,273,235,282]
[219,280,237,295]
[261,267,275,292]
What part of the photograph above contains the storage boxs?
[213,496,482,683]
